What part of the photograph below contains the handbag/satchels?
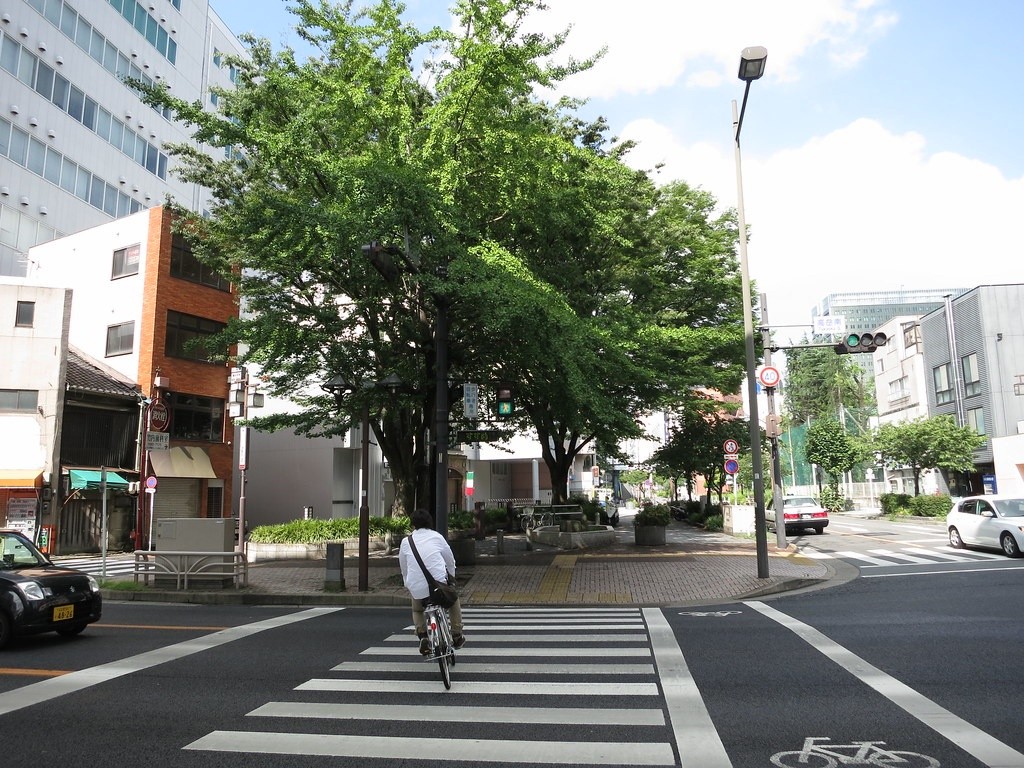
[430,580,457,609]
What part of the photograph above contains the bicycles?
[420,595,458,690]
[520,504,553,533]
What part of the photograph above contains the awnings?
[150,445,217,478]
[69,470,129,491]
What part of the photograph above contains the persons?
[507,501,517,533]
[399,510,466,653]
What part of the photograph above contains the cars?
[0,527,103,653]
[764,495,829,536]
[946,495,1024,559]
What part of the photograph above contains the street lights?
[228,372,264,562]
[731,46,770,576]
[320,370,402,591]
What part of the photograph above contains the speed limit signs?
[760,365,780,387]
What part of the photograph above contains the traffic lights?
[845,330,888,352]
[497,397,515,418]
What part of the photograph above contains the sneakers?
[454,636,465,650]
[419,637,430,656]
